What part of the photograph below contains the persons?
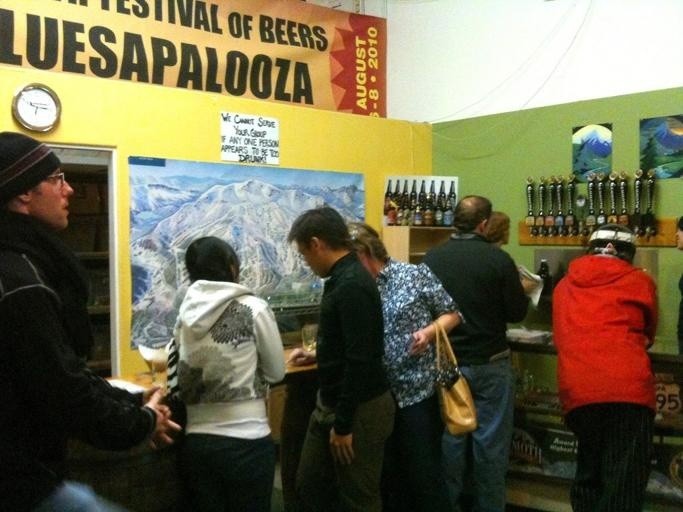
[1,132,183,511]
[344,222,466,512]
[551,224,659,512]
[287,208,395,511]
[672,216,683,417]
[420,196,530,511]
[168,237,287,511]
[484,212,538,294]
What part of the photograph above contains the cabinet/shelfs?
[382,224,461,272]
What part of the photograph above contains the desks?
[499,325,682,511]
[104,339,324,511]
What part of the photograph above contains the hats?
[0,132,61,204]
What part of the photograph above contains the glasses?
[42,168,67,185]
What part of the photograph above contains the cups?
[150,359,167,389]
[513,367,535,400]
[301,324,318,352]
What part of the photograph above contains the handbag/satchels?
[431,320,479,437]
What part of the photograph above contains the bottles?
[383,179,457,226]
[535,258,552,295]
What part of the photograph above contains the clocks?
[11,81,60,134]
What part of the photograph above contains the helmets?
[586,223,636,265]
